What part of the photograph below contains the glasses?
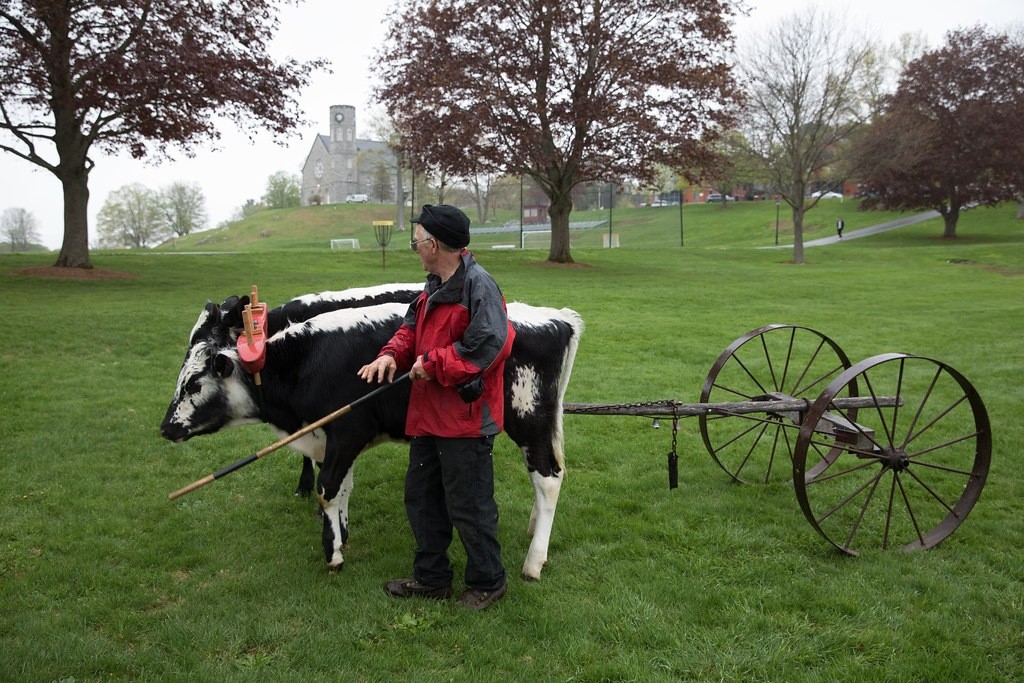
[410,236,437,251]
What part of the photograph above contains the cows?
[160,281,586,582]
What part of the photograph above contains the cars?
[706,194,735,202]
[640,200,669,207]
[811,190,844,200]
[346,194,369,204]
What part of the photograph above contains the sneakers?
[457,581,508,609]
[384,578,452,601]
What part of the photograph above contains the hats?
[409,204,471,249]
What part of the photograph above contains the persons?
[837,217,844,238]
[358,204,516,611]
